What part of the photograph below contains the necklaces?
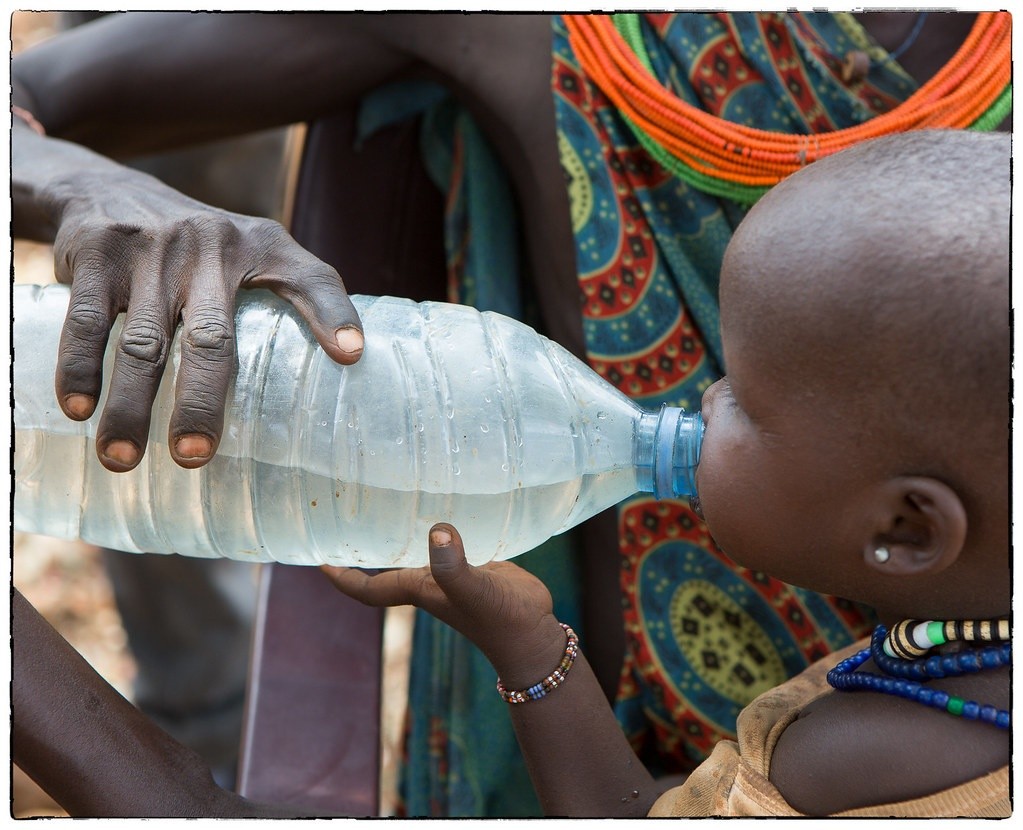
[826,617,1010,733]
[558,14,1011,200]
[785,12,930,82]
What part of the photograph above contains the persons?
[13,585,313,818]
[12,12,1012,818]
[319,131,1011,819]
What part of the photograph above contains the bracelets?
[496,623,578,705]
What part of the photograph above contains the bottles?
[13,282,705,568]
